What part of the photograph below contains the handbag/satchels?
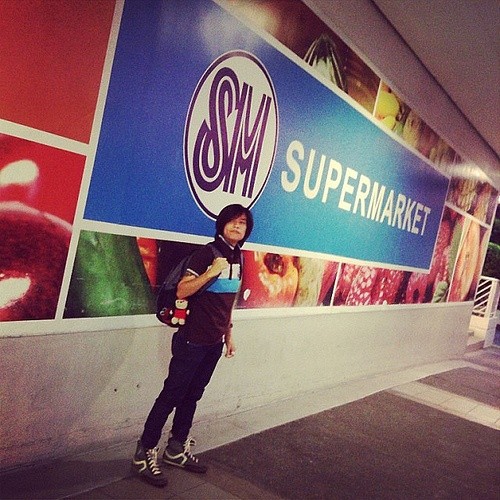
[156,246,221,327]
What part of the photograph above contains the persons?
[129,204,254,488]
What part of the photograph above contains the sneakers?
[162,434,209,472]
[130,438,167,486]
